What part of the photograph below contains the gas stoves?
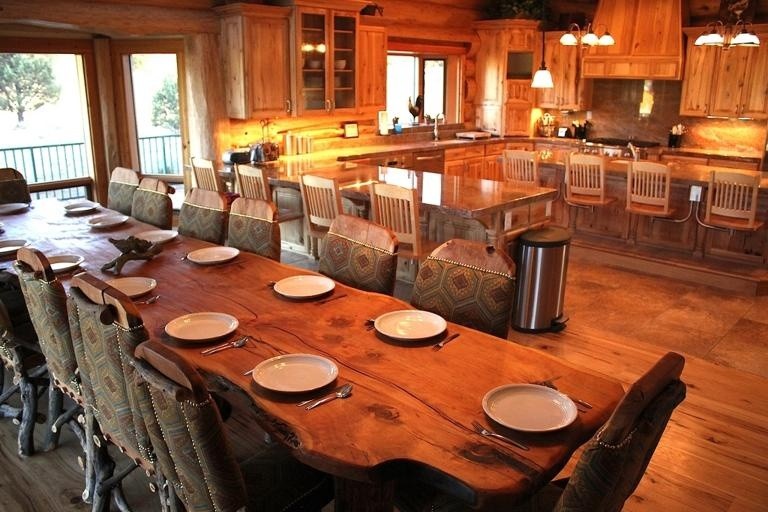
[580,136,661,161]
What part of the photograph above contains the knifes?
[200,338,239,354]
[296,382,350,406]
[316,292,347,304]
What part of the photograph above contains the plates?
[188,246,240,266]
[64,202,178,245]
[45,253,85,275]
[252,354,338,393]
[104,276,158,297]
[373,310,448,340]
[273,275,336,299]
[0,202,30,213]
[481,383,579,432]
[164,311,239,342]
[0,239,31,254]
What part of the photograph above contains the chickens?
[408,94,423,123]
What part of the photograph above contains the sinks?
[609,158,631,165]
[426,139,470,146]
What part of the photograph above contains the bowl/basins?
[302,59,346,68]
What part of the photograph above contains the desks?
[0,196,629,499]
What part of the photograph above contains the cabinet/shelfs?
[444,140,505,182]
[290,5,355,116]
[679,25,767,119]
[538,31,593,112]
[474,19,539,138]
[210,3,290,120]
[359,24,386,111]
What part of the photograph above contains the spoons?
[203,335,248,357]
[133,294,160,305]
[303,384,353,411]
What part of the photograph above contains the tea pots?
[248,143,267,165]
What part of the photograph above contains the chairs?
[522,350,687,512]
[695,170,764,233]
[12,246,94,506]
[122,337,335,511]
[64,271,169,511]
[624,161,694,224]
[0,267,48,460]
[0,167,32,204]
[503,148,561,202]
[563,152,618,213]
[107,156,517,341]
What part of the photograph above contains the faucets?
[433,112,448,140]
[626,141,642,161]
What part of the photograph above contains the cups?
[573,127,587,139]
[668,134,681,148]
[394,124,402,134]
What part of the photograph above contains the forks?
[469,419,530,451]
[434,332,458,352]
[543,377,592,408]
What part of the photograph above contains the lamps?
[560,22,615,46]
[693,0,760,47]
[530,32,554,89]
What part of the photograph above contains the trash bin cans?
[511,226,571,333]
